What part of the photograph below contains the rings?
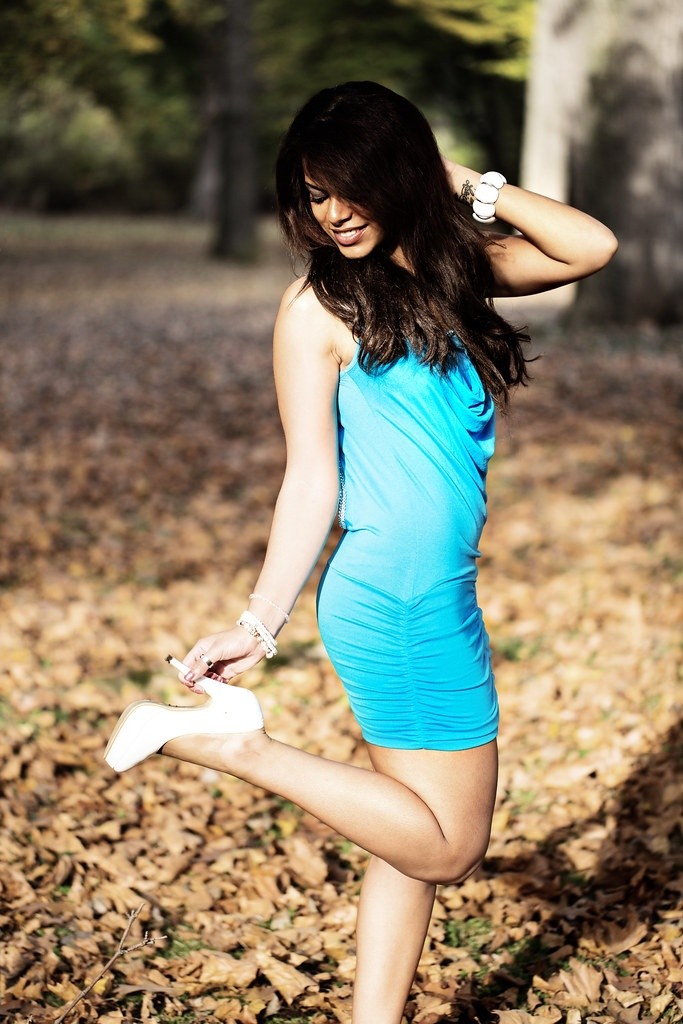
[201,654,214,670]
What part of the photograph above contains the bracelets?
[471,172,508,225]
[235,593,290,661]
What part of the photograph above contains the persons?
[107,83,620,1024]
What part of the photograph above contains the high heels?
[103,653,266,776]
